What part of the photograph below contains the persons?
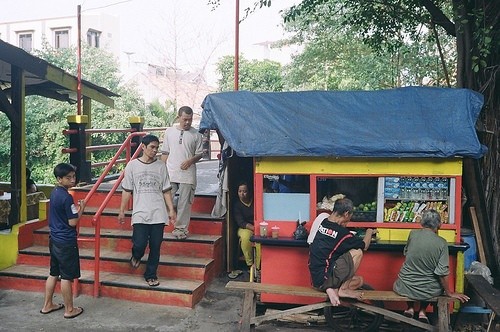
[157,106,204,238]
[308,198,381,306]
[39,163,84,319]
[233,181,261,273]
[26,168,38,193]
[263,178,295,193]
[117,134,176,285]
[392,209,470,322]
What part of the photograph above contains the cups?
[271,225,280,239]
[259,221,268,238]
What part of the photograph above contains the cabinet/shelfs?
[315,176,455,225]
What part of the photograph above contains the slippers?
[404,310,414,318]
[64,306,84,318]
[146,276,159,286]
[130,255,141,267]
[418,315,429,325]
[176,234,187,240]
[40,303,64,314]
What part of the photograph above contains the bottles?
[413,176,421,199]
[441,176,449,200]
[406,176,412,199]
[420,176,429,200]
[427,176,435,200]
[399,175,406,198]
[433,176,441,200]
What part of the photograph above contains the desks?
[0,190,47,229]
[250,234,471,312]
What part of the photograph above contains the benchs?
[462,267,500,332]
[224,280,457,331]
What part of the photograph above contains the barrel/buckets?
[461,229,477,274]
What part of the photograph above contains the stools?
[236,239,257,279]
